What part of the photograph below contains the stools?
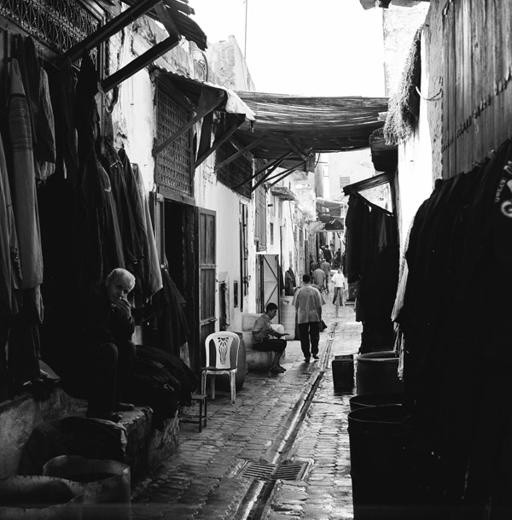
[182,393,208,432]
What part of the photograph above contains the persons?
[310,244,346,306]
[250,302,290,374]
[40,266,135,423]
[291,273,326,363]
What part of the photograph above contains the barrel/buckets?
[348,393,405,412]
[355,350,403,397]
[279,295,296,340]
[348,405,408,519]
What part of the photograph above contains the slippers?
[272,367,284,373]
[280,367,286,370]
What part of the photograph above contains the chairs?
[200,332,242,405]
[226,308,285,372]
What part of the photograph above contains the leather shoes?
[117,403,134,411]
